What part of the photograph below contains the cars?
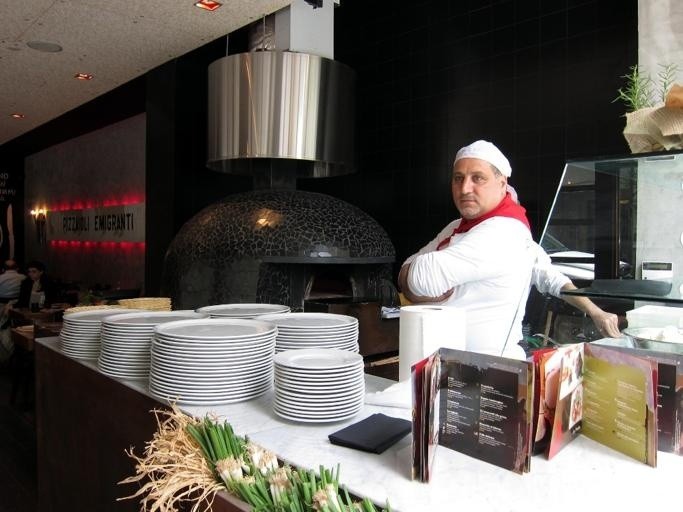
[526,232,630,309]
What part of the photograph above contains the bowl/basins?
[620,325,683,354]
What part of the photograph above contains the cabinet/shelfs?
[522,151,682,359]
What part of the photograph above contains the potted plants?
[611,62,683,153]
[82,291,109,306]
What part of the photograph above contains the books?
[411,342,683,483]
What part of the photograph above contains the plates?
[252,312,360,384]
[272,348,365,425]
[196,303,292,320]
[59,308,153,362]
[148,318,279,407]
[96,309,212,381]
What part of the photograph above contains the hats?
[453,140,512,177]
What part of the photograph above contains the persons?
[398,140,533,362]
[506,184,624,337]
[4,261,52,315]
[0,259,27,304]
